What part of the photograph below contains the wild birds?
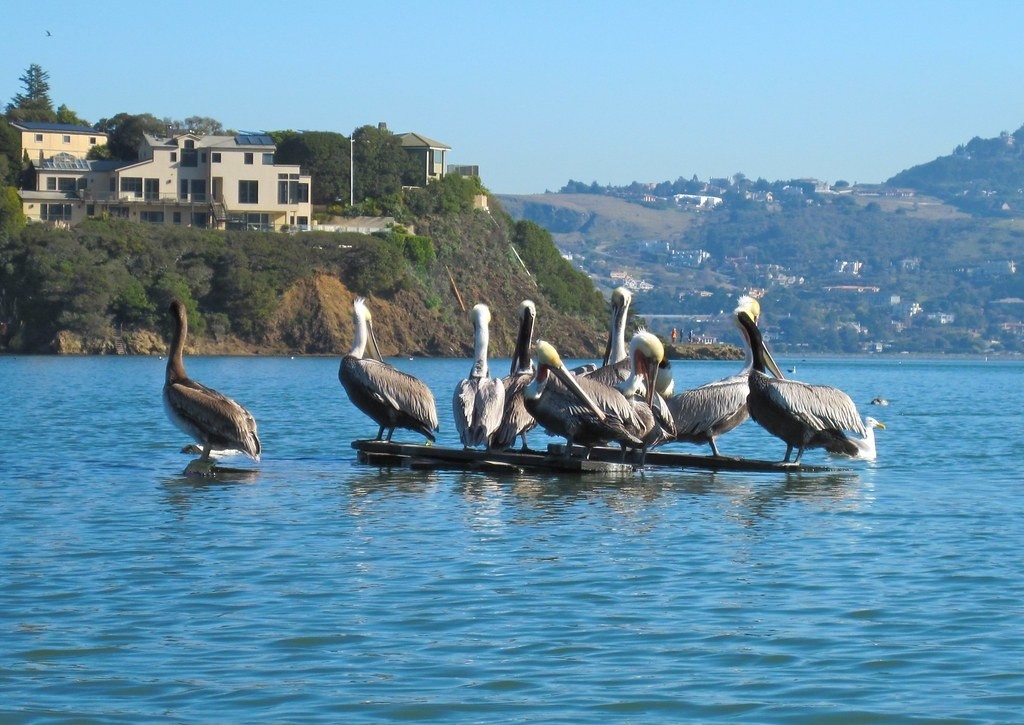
[666,294,867,466]
[833,416,886,460]
[523,285,679,467]
[161,300,260,467]
[452,303,506,449]
[871,398,888,406]
[488,299,539,452]
[337,295,440,444]
